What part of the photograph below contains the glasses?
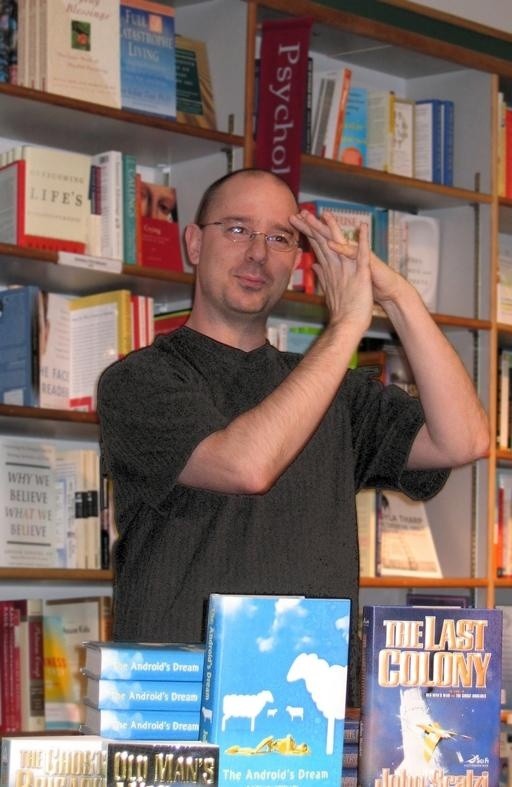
[202,222,303,252]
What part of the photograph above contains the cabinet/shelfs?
[4,2,253,738]
[244,1,509,722]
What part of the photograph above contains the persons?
[94,167,493,644]
